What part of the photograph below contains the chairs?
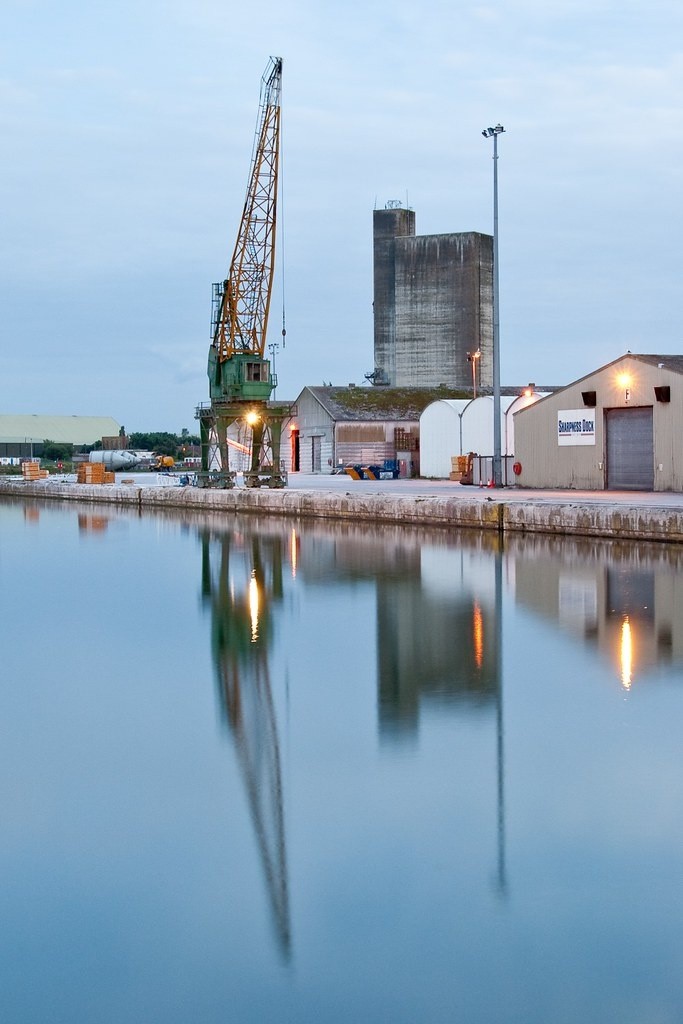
[361,466,401,480]
[344,466,369,479]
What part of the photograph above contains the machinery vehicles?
[149,456,175,473]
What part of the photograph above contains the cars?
[174,463,185,469]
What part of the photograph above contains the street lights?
[191,446,194,457]
[467,350,480,398]
[482,126,507,486]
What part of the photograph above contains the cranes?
[198,54,289,488]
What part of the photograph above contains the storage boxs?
[450,456,473,480]
[77,463,115,484]
[22,462,49,480]
